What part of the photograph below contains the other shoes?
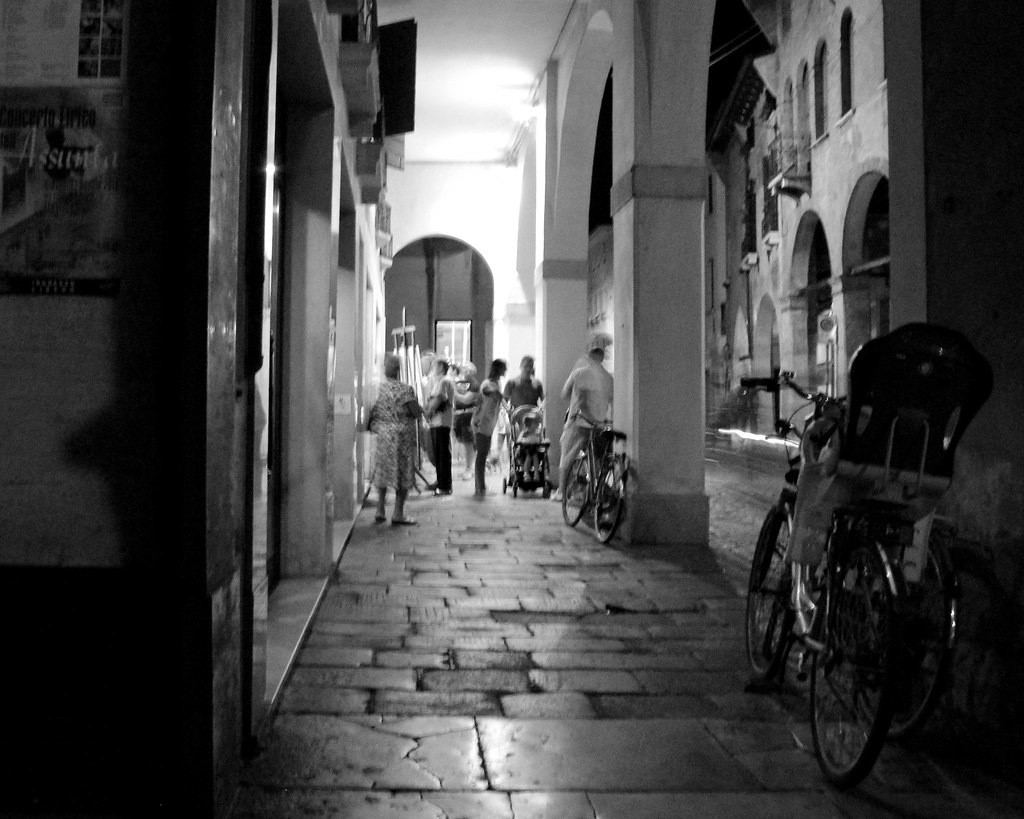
[426,482,440,490]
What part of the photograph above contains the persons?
[470,358,506,500]
[454,360,480,482]
[550,347,613,501]
[501,355,546,425]
[491,394,512,469]
[420,353,460,495]
[367,355,422,526]
[515,413,544,483]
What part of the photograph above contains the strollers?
[506,400,553,500]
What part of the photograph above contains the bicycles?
[562,407,638,543]
[739,317,995,782]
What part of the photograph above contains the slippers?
[391,516,417,525]
[375,515,387,523]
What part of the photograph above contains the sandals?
[534,473,540,481]
[524,472,531,482]
